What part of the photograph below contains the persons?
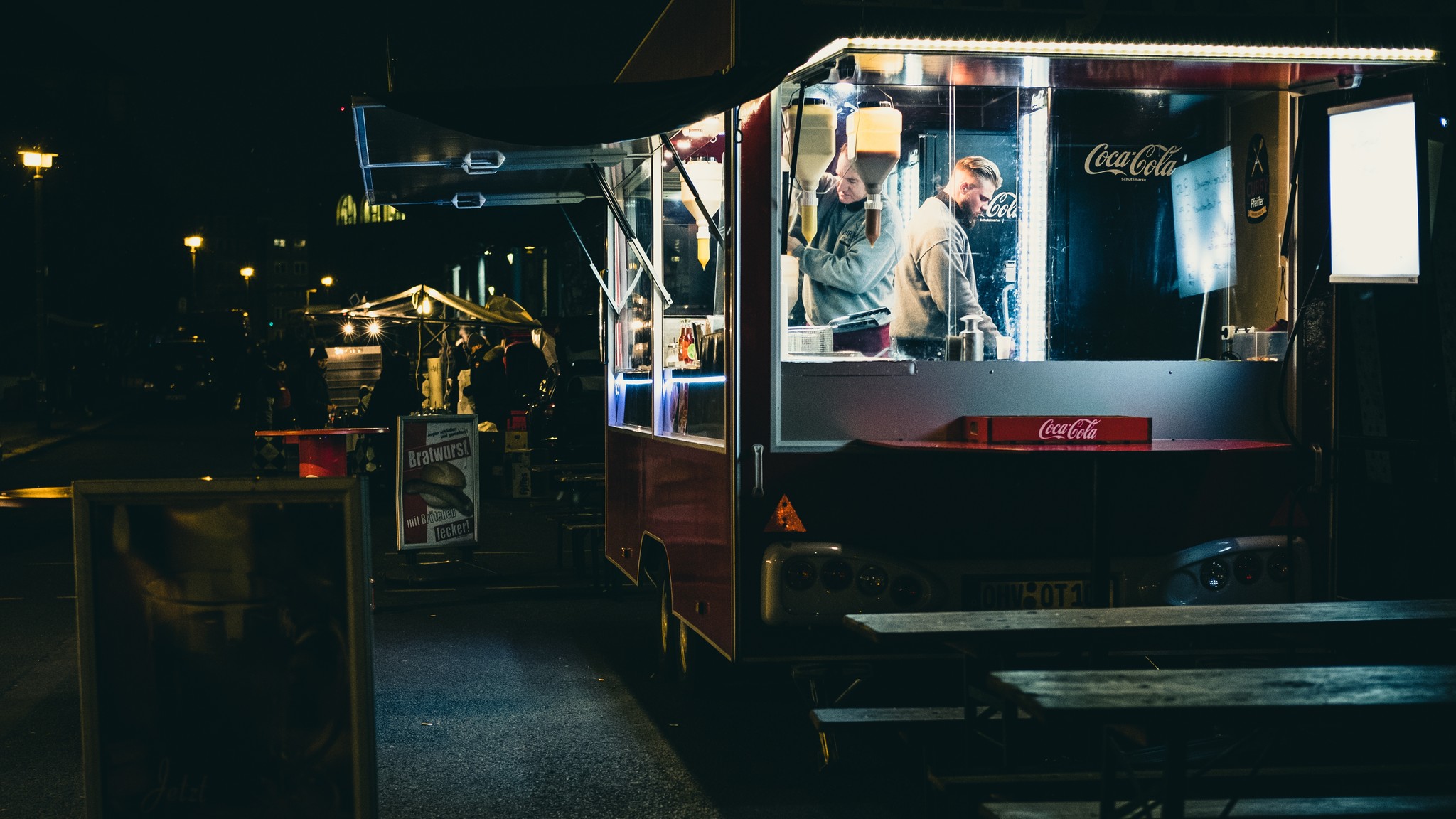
[442,323,540,494]
[887,157,1011,361]
[779,140,898,359]
[230,342,375,478]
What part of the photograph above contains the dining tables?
[253,426,390,478]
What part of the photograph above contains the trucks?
[346,0,1446,703]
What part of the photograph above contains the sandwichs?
[403,460,475,517]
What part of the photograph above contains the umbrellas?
[289,282,544,408]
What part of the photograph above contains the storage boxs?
[504,410,528,457]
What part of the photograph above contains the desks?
[528,462,606,557]
[555,475,607,578]
[843,596,1456,796]
[989,664,1455,819]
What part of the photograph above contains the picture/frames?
[69,473,377,819]
[395,413,480,552]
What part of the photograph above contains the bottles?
[683,317,694,364]
[677,320,686,361]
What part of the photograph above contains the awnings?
[343,50,803,151]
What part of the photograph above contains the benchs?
[528,496,606,544]
[541,512,606,578]
[527,500,606,559]
[977,794,1456,819]
[809,707,1456,818]
[563,523,624,582]
[924,765,1455,818]
